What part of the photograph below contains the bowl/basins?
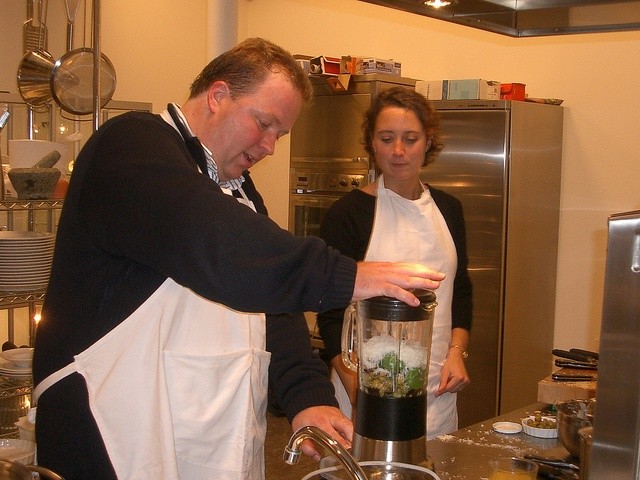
[0,346,37,369]
[1,435,40,468]
[554,397,596,457]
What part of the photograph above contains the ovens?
[284,169,427,256]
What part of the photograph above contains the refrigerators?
[419,98,564,431]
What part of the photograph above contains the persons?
[316,86,473,443]
[32,37,446,480]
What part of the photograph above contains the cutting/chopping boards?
[536,352,598,406]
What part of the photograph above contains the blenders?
[340,285,439,473]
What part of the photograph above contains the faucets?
[281,424,372,480]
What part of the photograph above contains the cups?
[485,454,539,479]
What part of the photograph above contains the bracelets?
[449,344,469,358]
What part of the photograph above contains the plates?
[521,413,563,439]
[0,365,37,379]
[527,97,565,107]
[0,227,60,296]
